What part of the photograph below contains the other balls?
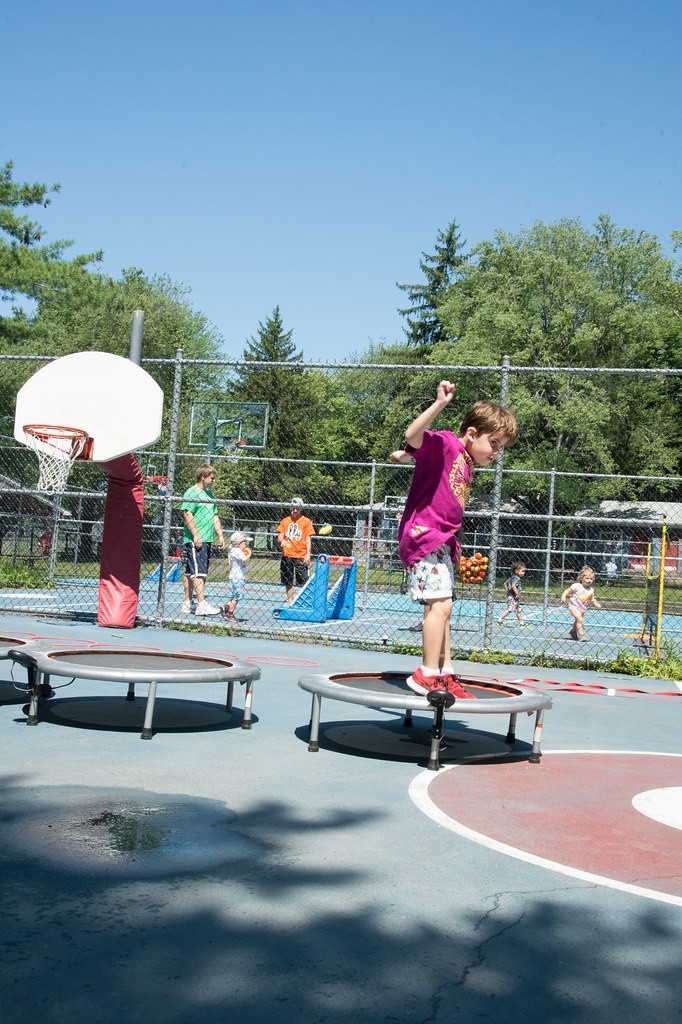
[242,547,251,557]
[456,551,488,584]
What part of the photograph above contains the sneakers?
[405,668,446,695]
[195,603,220,615]
[220,604,230,618]
[224,613,239,622]
[182,601,197,614]
[444,674,476,699]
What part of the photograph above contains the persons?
[277,497,316,605]
[388,380,518,699]
[180,464,225,616]
[495,561,529,629]
[561,565,601,642]
[221,531,253,622]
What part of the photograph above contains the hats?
[292,498,304,504]
[230,531,254,544]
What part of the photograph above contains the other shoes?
[577,636,587,641]
[495,621,503,628]
[570,630,577,639]
[520,623,529,628]
